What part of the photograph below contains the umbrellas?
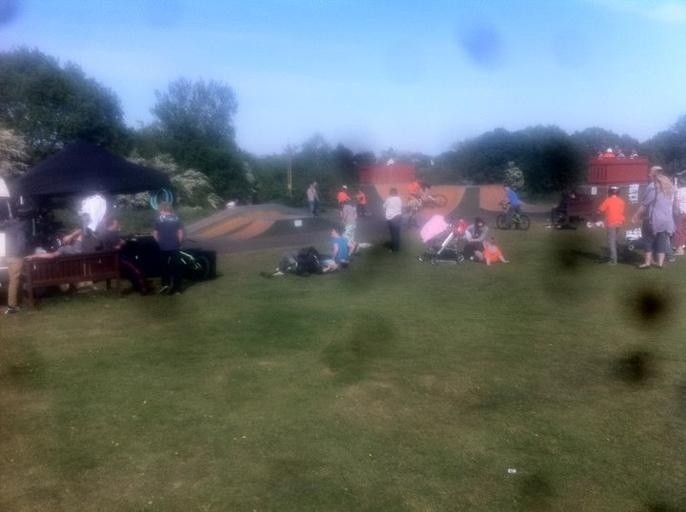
[14,138,167,228]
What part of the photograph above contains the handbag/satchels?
[296,247,321,274]
[643,218,653,235]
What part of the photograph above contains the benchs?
[20,248,121,307]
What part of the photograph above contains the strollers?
[423,218,468,265]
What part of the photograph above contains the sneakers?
[160,285,168,293]
[8,307,20,312]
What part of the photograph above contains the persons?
[503,184,521,225]
[103,217,137,269]
[632,166,686,270]
[596,186,627,267]
[24,216,95,261]
[1,211,25,316]
[384,178,423,255]
[463,218,511,265]
[306,181,366,268]
[151,203,185,293]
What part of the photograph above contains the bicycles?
[495,201,530,231]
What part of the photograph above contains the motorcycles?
[403,191,448,227]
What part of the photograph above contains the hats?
[609,186,620,190]
